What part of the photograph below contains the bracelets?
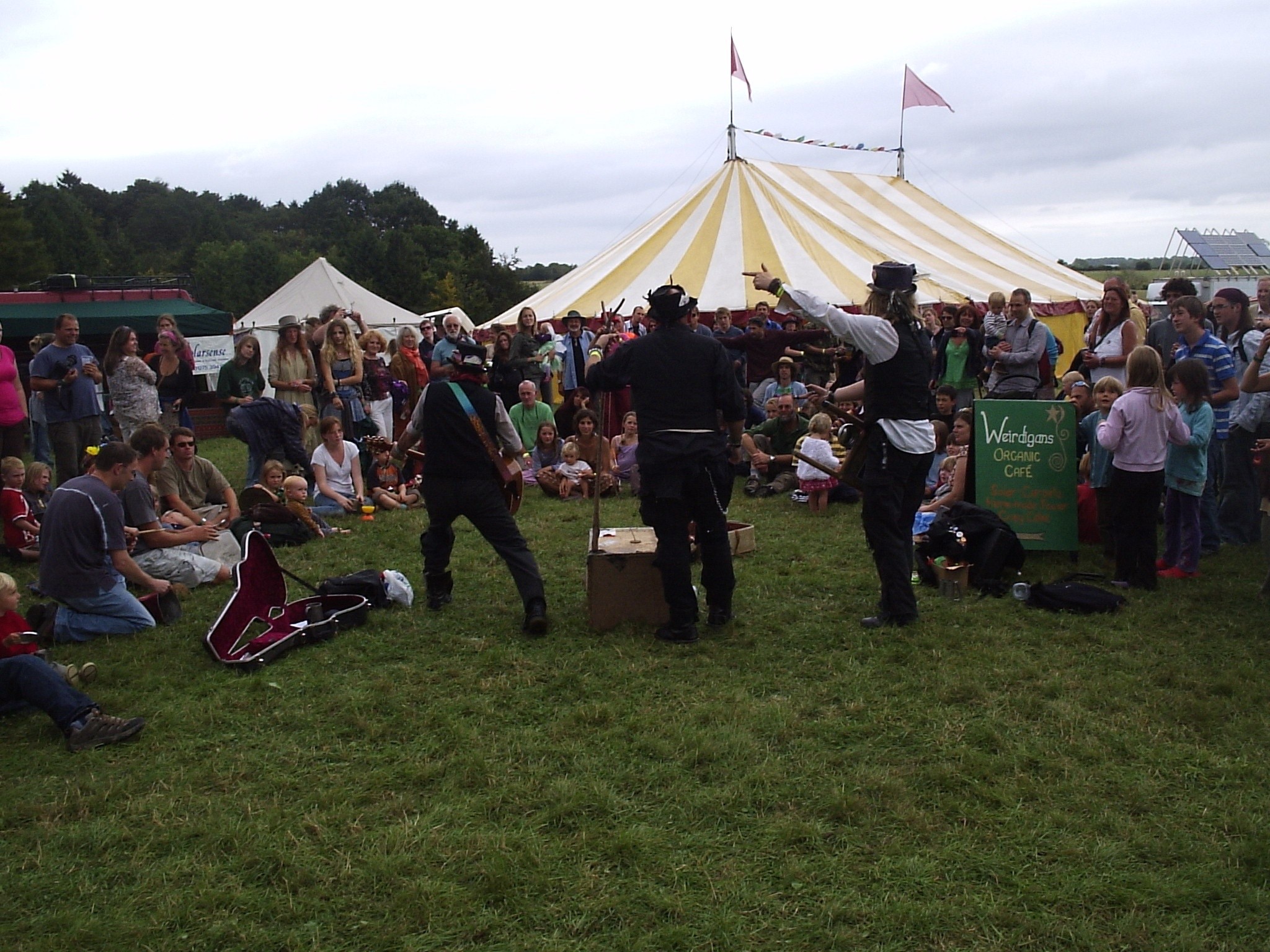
[800,351,805,356]
[524,452,531,459]
[611,466,616,468]
[1100,357,1106,367]
[591,351,601,361]
[776,286,786,297]
[587,344,602,352]
[616,338,622,343]
[337,378,341,385]
[823,348,827,355]
[768,278,782,297]
[594,473,596,477]
[587,347,603,358]
[233,397,239,404]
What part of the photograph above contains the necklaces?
[162,356,177,376]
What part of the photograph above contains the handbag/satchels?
[381,569,415,607]
[488,369,506,391]
[319,569,392,609]
[1025,578,1128,616]
[231,513,314,548]
[915,500,1025,598]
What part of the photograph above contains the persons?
[0,262,1270,755]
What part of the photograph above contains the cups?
[305,602,324,624]
[81,356,93,373]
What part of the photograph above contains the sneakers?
[66,707,145,750]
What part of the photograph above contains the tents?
[0,161,1140,397]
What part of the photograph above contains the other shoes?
[745,475,776,497]
[860,616,918,628]
[543,376,552,382]
[707,611,733,624]
[1155,558,1200,578]
[425,593,452,612]
[1110,581,1131,590]
[655,624,700,642]
[64,661,98,687]
[523,615,548,635]
[992,362,1010,374]
[35,601,58,638]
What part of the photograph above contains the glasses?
[778,404,791,409]
[334,331,343,334]
[177,441,195,447]
[958,407,970,412]
[1071,381,1090,390]
[941,316,953,320]
[1010,304,1022,306]
[1211,304,1227,310]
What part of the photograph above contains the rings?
[527,461,530,464]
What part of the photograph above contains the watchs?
[197,518,206,525]
[768,455,776,464]
[1254,352,1264,363]
[825,392,835,405]
[330,391,337,397]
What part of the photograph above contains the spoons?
[216,519,227,527]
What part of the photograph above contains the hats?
[445,341,488,373]
[866,261,917,295]
[597,312,621,323]
[772,357,800,378]
[278,315,302,334]
[646,284,698,321]
[562,310,587,327]
[781,315,799,327]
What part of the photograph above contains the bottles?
[1013,583,1031,601]
